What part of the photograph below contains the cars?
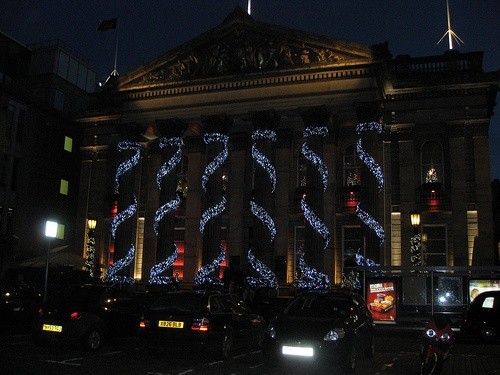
[138,290,268,363]
[262,288,375,374]
[33,281,147,353]
[0,266,44,333]
[460,291,500,344]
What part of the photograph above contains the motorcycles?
[417,312,467,375]
